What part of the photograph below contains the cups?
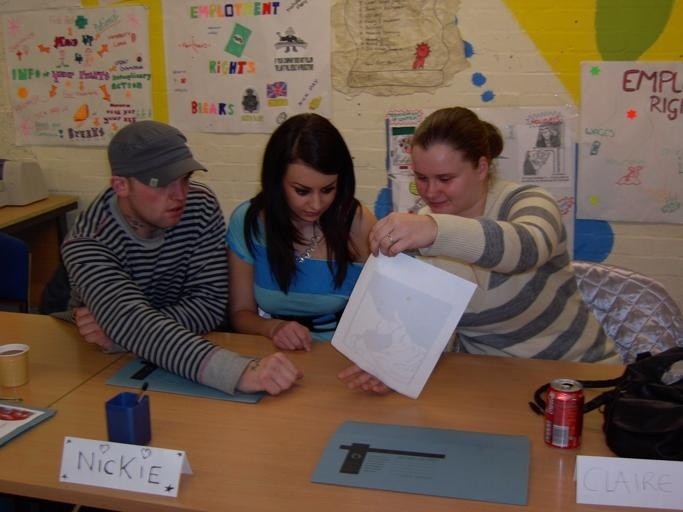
[106,392,150,445]
[1,344,30,387]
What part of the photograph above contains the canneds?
[543,377,584,450]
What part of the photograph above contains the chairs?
[0,230,33,313]
[569,259,683,385]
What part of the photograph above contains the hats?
[108,121,208,189]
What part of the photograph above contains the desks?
[0,194,80,315]
[0,332,683,512]
[0,310,128,410]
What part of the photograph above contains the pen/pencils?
[133,383,149,406]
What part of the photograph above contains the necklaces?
[296,224,322,264]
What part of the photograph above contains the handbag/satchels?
[604,348,683,461]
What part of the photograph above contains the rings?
[384,233,394,244]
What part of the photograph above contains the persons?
[336,107,622,393]
[59,121,304,396]
[225,114,377,352]
